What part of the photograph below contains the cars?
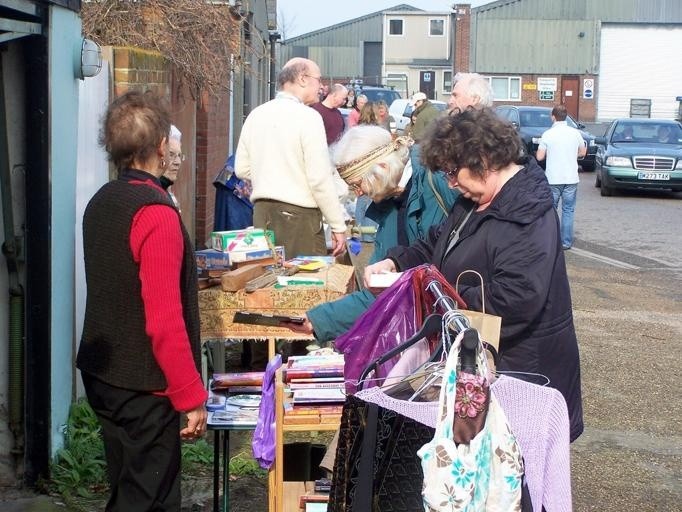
[356,86,400,112]
[492,104,597,172]
[593,117,682,198]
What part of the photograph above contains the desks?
[204,378,263,512]
[197,259,357,395]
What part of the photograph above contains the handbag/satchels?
[417,328,524,512]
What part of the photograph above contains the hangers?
[339,280,551,402]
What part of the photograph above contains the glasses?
[441,161,467,183]
[169,152,186,161]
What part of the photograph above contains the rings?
[196,427,202,431]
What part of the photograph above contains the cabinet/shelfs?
[266,363,341,512]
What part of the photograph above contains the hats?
[408,92,428,107]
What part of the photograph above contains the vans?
[388,97,448,141]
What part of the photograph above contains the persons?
[157,123,184,215]
[653,125,678,142]
[73,90,208,512]
[615,126,635,140]
